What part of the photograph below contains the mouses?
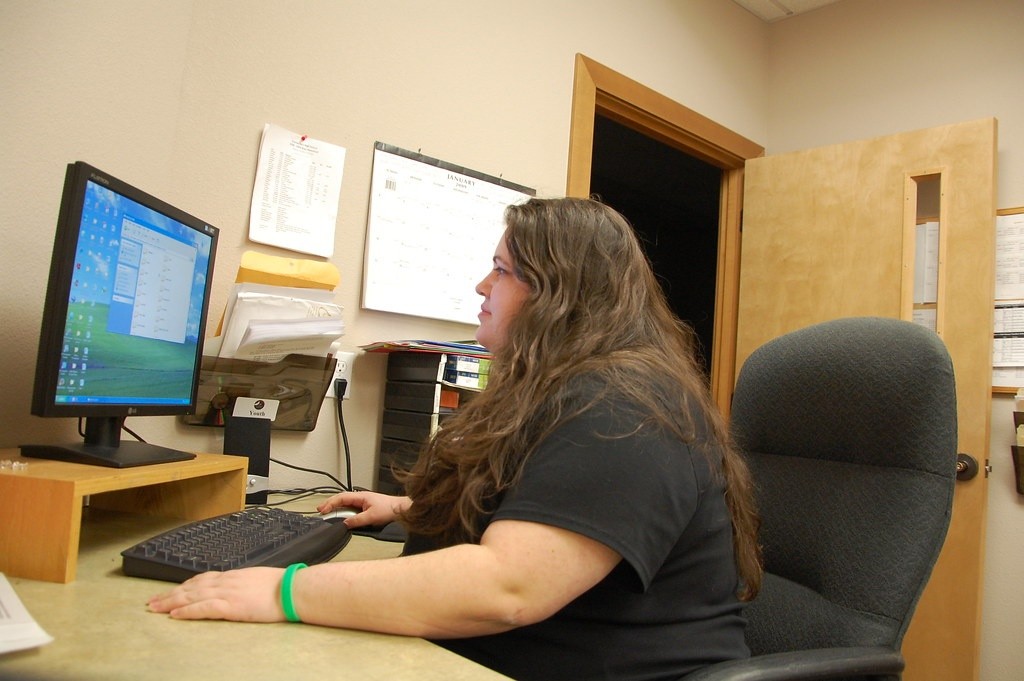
[312,508,360,526]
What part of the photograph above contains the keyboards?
[121,504,351,586]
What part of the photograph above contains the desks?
[0,491,531,681]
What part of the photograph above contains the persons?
[147,194,763,681]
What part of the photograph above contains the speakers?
[223,416,271,505]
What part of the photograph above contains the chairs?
[677,318,958,681]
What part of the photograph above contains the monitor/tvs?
[17,161,219,471]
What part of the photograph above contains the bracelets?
[280,563,308,623]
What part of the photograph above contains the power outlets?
[324,352,355,399]
